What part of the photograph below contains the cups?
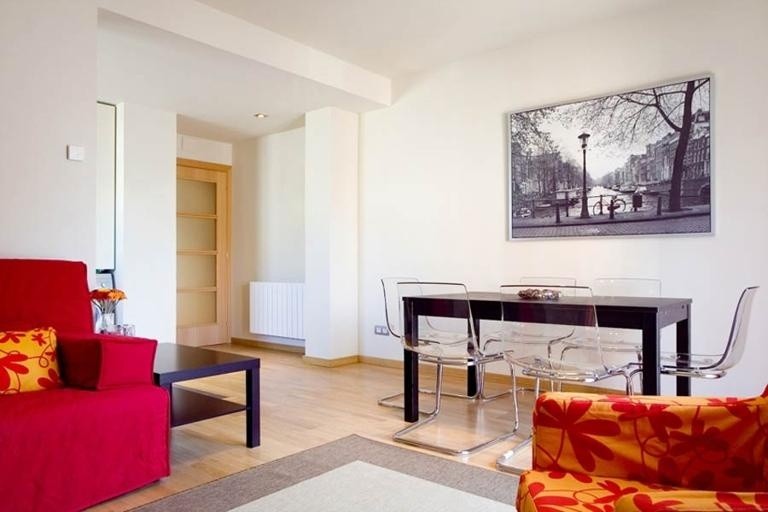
[105,323,136,337]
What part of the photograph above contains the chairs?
[496,286,633,473]
[481,277,661,400]
[380,274,518,456]
[628,286,759,383]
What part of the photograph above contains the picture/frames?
[505,73,719,239]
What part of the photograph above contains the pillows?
[1,325,64,396]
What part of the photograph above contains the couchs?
[1,256,173,508]
[513,389,768,512]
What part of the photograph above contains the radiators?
[248,280,306,340]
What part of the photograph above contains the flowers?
[90,287,125,316]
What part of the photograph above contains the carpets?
[121,434,522,512]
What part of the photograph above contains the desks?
[393,289,693,425]
